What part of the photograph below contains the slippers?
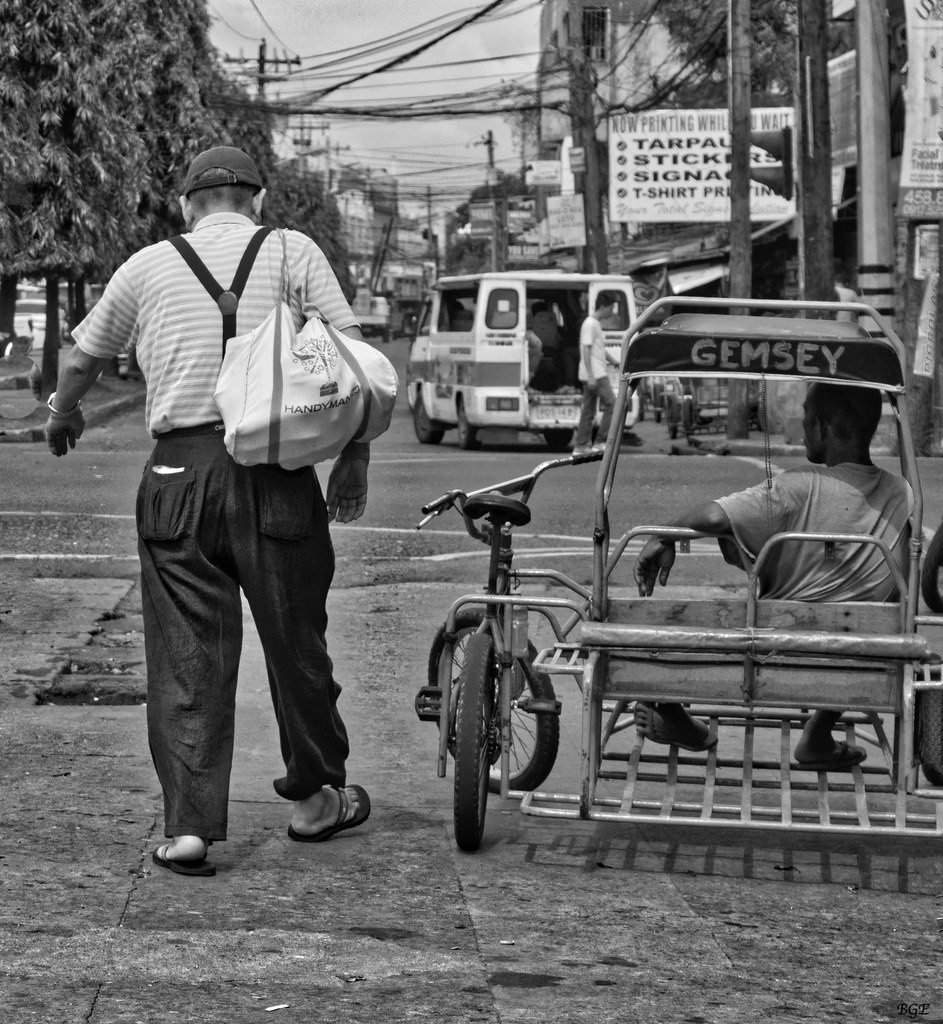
[797,740,867,770]
[634,700,719,752]
[152,843,216,876]
[288,784,371,843]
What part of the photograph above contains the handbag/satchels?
[212,227,399,470]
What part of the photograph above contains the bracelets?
[47,393,81,418]
[614,363,619,367]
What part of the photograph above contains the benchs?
[605,524,908,707]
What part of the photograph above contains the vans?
[402,269,638,450]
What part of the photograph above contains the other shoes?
[593,441,607,451]
[572,444,597,456]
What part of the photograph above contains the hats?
[185,147,264,201]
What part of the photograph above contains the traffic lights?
[749,124,794,202]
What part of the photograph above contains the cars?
[15,297,100,349]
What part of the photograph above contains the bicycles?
[413,440,614,856]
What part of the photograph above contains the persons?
[833,282,859,324]
[633,381,917,768]
[451,293,621,453]
[44,149,372,874]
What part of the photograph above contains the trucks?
[355,295,392,343]
